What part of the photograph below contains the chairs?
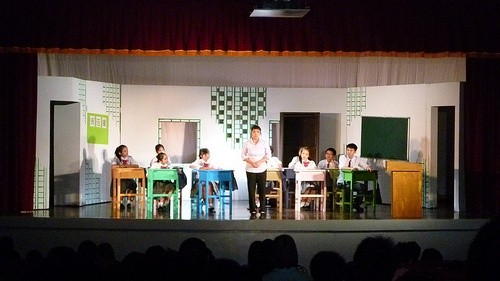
[190,170,201,208]
[173,167,183,207]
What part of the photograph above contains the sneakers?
[352,201,364,212]
[251,212,256,219]
[120,203,125,210]
[127,203,132,209]
[301,205,310,210]
[259,213,266,219]
[158,205,169,212]
[208,207,216,214]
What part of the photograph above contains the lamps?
[249,0,311,18]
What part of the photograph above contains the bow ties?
[204,162,209,167]
[122,160,129,165]
[303,162,309,167]
[161,165,168,169]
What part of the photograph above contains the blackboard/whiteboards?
[360,116,410,162]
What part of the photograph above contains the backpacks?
[173,167,187,189]
[324,168,334,192]
[364,170,382,206]
[278,168,289,192]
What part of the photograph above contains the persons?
[241,125,272,219]
[110,145,139,209]
[189,148,216,213]
[0,219,500,281]
[288,147,303,169]
[256,146,283,207]
[293,147,319,211]
[150,144,172,170]
[318,148,339,169]
[337,143,372,213]
[146,152,174,212]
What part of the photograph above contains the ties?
[327,163,329,169]
[348,160,351,168]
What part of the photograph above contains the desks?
[340,170,378,213]
[198,169,234,216]
[293,169,326,212]
[253,168,283,212]
[148,169,178,213]
[322,168,342,212]
[112,167,145,210]
[284,168,313,208]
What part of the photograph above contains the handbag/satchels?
[110,179,127,197]
[220,171,238,191]
